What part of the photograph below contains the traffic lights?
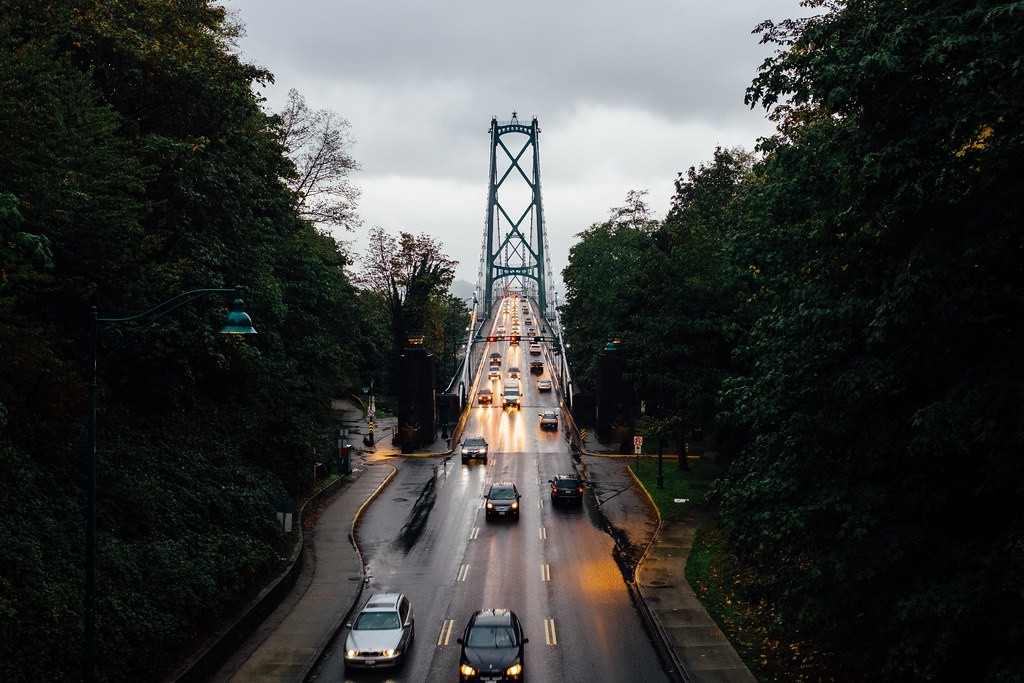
[487,336,497,342]
[510,336,521,341]
[531,336,544,341]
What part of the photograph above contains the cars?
[539,411,559,431]
[483,482,522,523]
[509,341,520,346]
[537,378,553,392]
[489,352,502,366]
[507,367,521,380]
[477,388,493,404]
[488,365,501,380]
[460,436,488,465]
[344,593,416,669]
[547,474,585,506]
[456,607,529,683]
[529,359,544,374]
[529,344,541,355]
[497,293,536,336]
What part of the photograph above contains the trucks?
[501,378,523,411]
